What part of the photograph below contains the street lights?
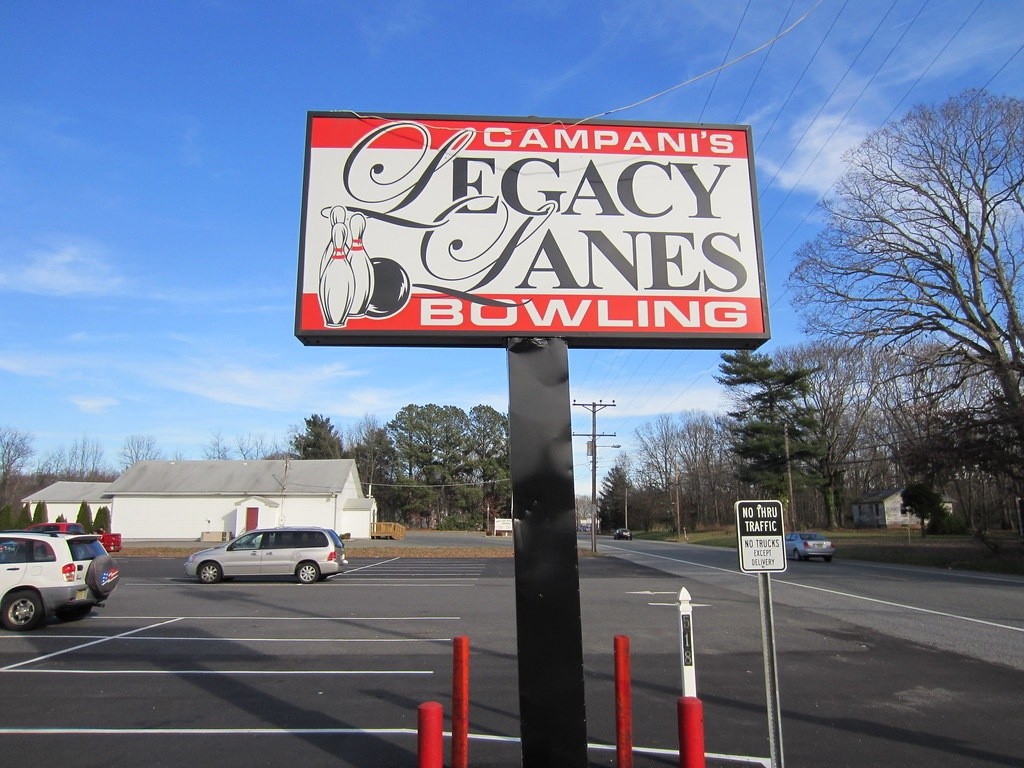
[592,445,622,552]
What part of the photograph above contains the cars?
[0,540,17,553]
[785,532,835,562]
[613,528,632,540]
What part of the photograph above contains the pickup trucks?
[12,523,121,561]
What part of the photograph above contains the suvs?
[184,526,349,584]
[0,530,120,630]
